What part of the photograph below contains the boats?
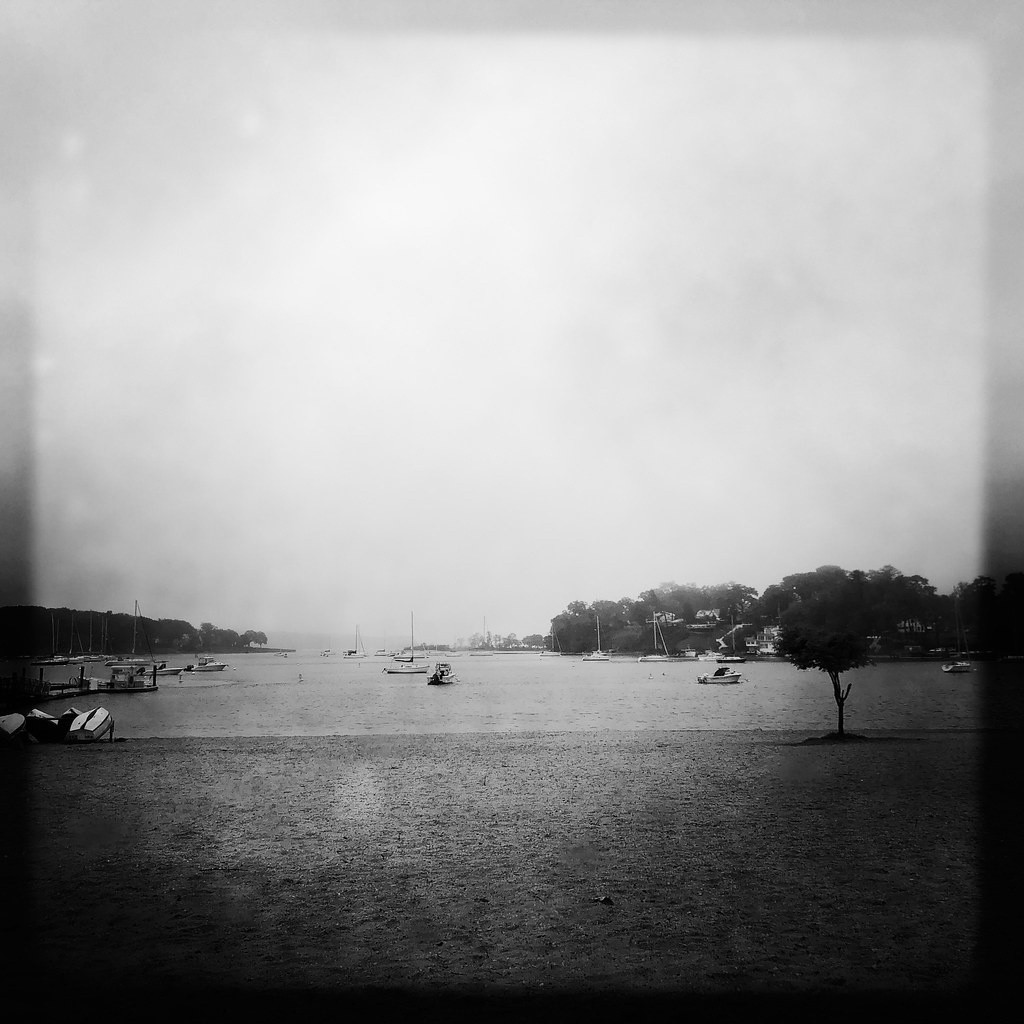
[426,663,455,685]
[275,651,283,656]
[697,650,722,660]
[680,648,699,657]
[393,650,425,658]
[69,706,113,742]
[183,657,226,672]
[0,713,26,742]
[136,664,184,676]
[697,667,742,684]
[374,649,386,655]
[446,651,463,657]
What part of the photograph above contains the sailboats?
[941,605,971,672]
[716,615,746,663]
[426,644,443,656]
[104,600,166,666]
[540,626,561,656]
[469,616,493,656]
[639,611,670,662]
[583,616,609,661]
[31,614,115,665]
[384,611,430,674]
[343,626,363,659]
